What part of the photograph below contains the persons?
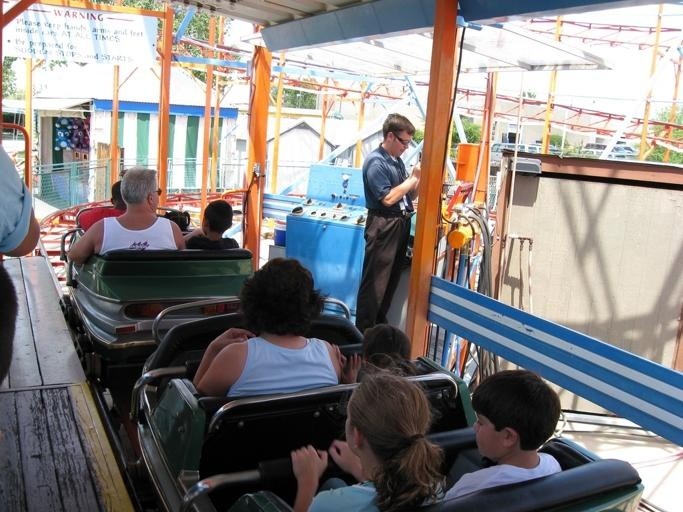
[445,370,562,502]
[191,257,341,398]
[66,166,239,263]
[355,112,421,336]
[0,143,40,384]
[332,324,426,384]
[290,373,445,512]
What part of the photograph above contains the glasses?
[154,188,161,195]
[394,134,411,145]
